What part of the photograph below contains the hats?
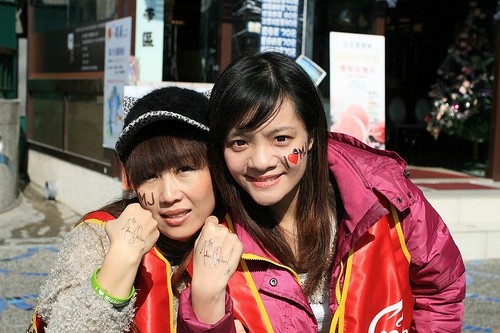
[116,86,210,159]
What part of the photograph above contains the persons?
[176,51,467,333]
[28,87,224,333]
